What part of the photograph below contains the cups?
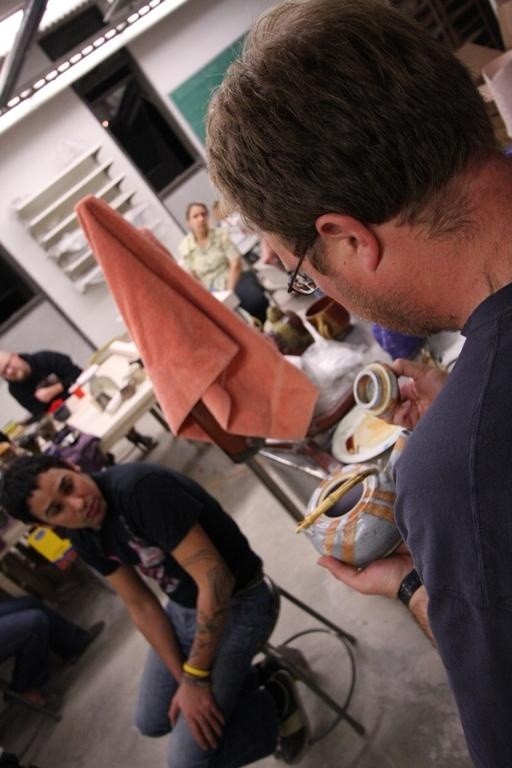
[303,294,355,342]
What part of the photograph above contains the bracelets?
[399,566,424,611]
[182,661,211,680]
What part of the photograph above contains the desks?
[1,289,242,598]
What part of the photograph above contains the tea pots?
[291,429,413,570]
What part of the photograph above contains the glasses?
[286,231,322,296]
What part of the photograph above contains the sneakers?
[69,620,108,670]
[267,667,312,766]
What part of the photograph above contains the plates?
[329,398,406,464]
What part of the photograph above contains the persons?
[0,456,310,767]
[205,0,511,768]
[0,594,106,722]
[176,198,269,327]
[0,349,159,469]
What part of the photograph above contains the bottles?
[264,306,313,356]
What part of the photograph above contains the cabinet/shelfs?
[12,143,159,291]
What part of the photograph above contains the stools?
[234,566,369,747]
